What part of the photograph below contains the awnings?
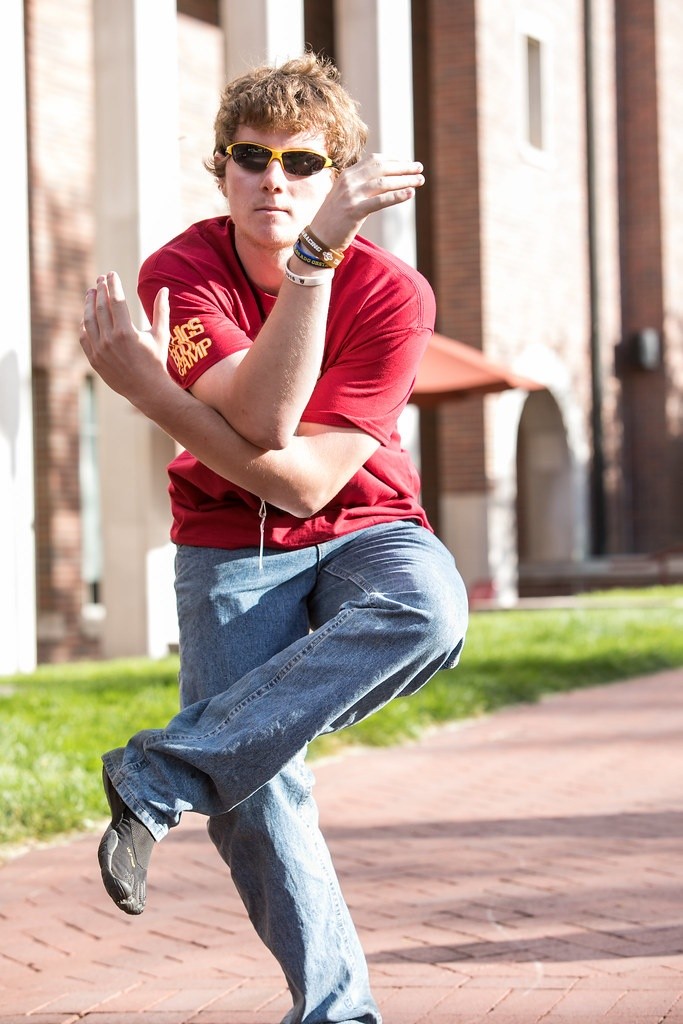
[405,335,546,408]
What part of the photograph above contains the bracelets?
[283,256,335,287]
[299,225,345,269]
[293,238,334,269]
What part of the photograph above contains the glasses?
[224,141,339,176]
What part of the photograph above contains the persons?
[76,49,472,1024]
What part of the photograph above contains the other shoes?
[102,763,154,916]
